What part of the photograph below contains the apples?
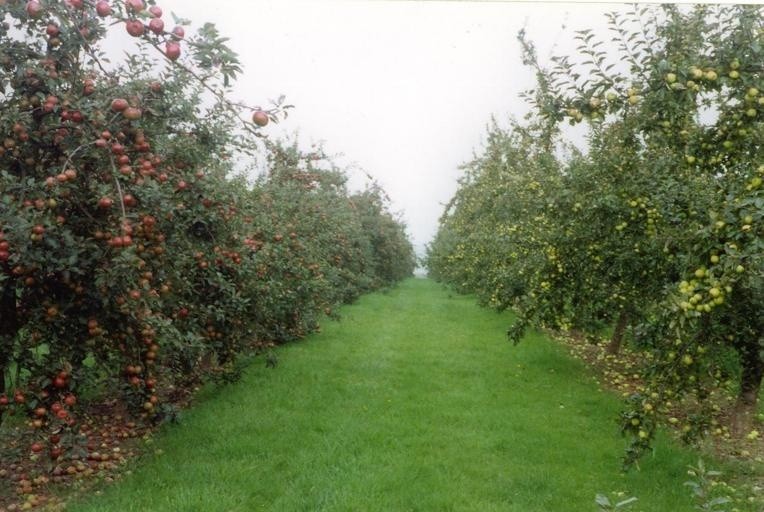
[0,0,764,512]
